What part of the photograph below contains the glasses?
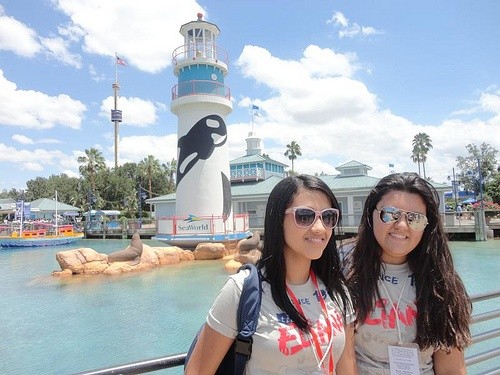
[376,206,429,230]
[282,206,339,229]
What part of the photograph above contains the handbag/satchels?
[184,264,260,375]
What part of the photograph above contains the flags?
[253,105,259,109]
[117,56,126,66]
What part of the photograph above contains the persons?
[445,203,455,210]
[186,174,358,375]
[462,205,467,219]
[8,217,51,231]
[340,172,474,374]
[456,203,463,220]
[467,202,472,220]
